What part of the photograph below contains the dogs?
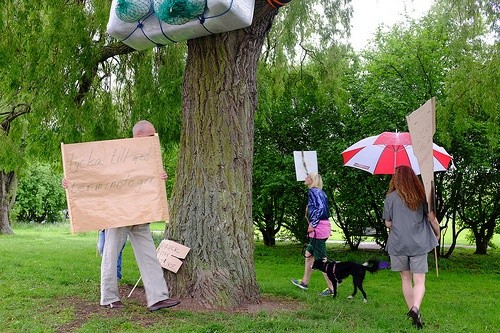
[310,257,381,304]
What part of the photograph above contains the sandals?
[407,306,422,329]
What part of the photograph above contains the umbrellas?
[340,128,454,176]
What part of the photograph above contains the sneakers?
[291,278,309,290]
[318,288,336,297]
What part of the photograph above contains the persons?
[290,172,339,296]
[61,119,182,312]
[382,165,440,330]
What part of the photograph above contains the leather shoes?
[149,298,181,311]
[110,301,125,308]
